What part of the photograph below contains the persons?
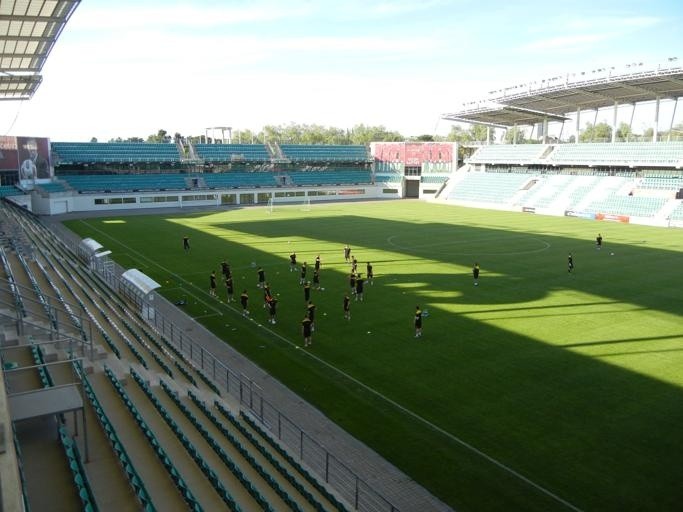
[567,253,574,273]
[472,262,480,286]
[208,243,373,348]
[181,234,192,256]
[21,139,50,179]
[596,233,602,250]
[413,305,422,338]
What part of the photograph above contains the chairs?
[437,143,682,220]
[91,324,226,396]
[78,394,351,510]
[90,271,146,324]
[0,139,373,201]
[0,202,91,512]
